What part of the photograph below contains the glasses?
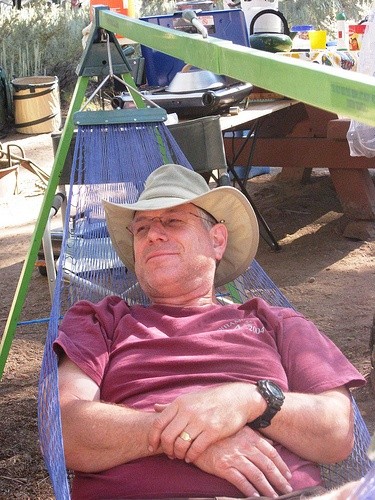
[125,209,215,238]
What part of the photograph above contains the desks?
[160,102,302,252]
[176,50,375,221]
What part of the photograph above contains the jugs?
[248,9,297,53]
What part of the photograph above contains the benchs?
[327,118,375,139]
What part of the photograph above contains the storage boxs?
[38,210,128,276]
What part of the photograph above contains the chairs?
[0,143,55,194]
[42,115,228,307]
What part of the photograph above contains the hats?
[101,164,260,288]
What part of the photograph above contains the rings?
[180,432,192,442]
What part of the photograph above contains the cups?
[336,12,347,51]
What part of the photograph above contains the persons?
[350,37,359,50]
[37,163,366,500]
[246,379,286,430]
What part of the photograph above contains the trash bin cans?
[11,76,61,134]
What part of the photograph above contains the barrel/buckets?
[10,75,62,134]
[309,31,326,51]
